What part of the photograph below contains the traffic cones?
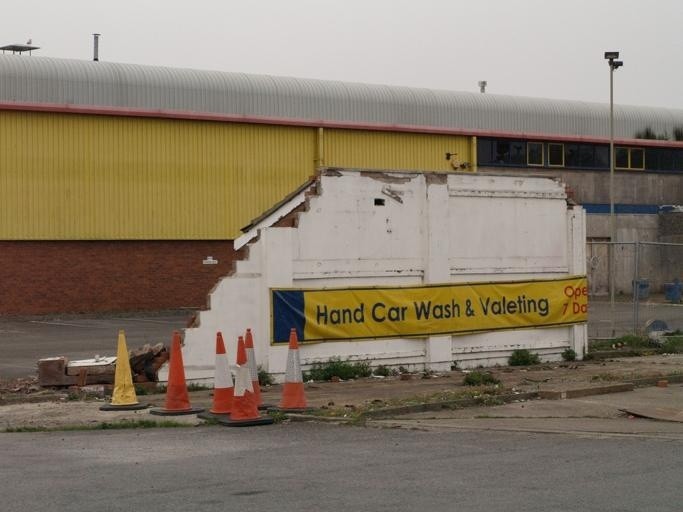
[263,327,319,414]
[98,326,152,413]
[146,328,207,418]
[216,334,274,428]
[193,331,236,421]
[244,327,272,410]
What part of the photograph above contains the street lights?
[603,51,624,240]
